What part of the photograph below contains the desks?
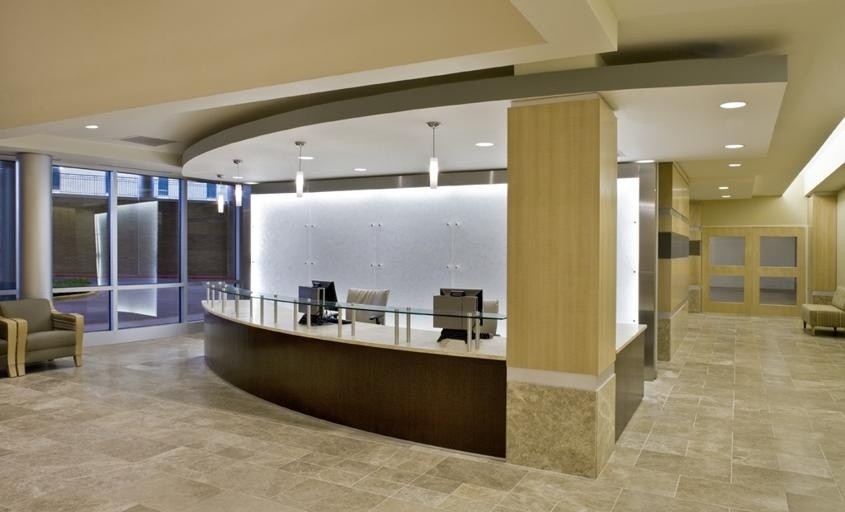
[811,289,834,304]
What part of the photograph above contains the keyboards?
[326,317,352,324]
[472,333,493,339]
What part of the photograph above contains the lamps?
[295,141,305,197]
[233,159,242,206]
[217,174,225,214]
[427,121,440,189]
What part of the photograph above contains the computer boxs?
[299,286,325,315]
[433,295,478,330]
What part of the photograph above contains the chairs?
[800,286,845,335]
[473,300,500,336]
[346,288,390,325]
[0,316,18,378]
[0,297,84,377]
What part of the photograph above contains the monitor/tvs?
[437,288,483,344]
[299,280,337,325]
